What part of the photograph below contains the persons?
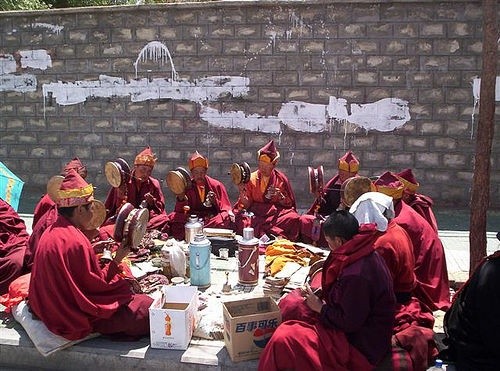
[257,212,396,371]
[306,150,450,309]
[443,232,500,371]
[309,177,417,303]
[0,157,155,342]
[99,149,172,241]
[168,153,235,239]
[234,141,300,239]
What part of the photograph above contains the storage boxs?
[221,295,283,363]
[148,286,197,351]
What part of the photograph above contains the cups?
[219,248,229,260]
[171,276,184,286]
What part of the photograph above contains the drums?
[308,165,324,195]
[340,177,375,208]
[168,168,193,195]
[84,198,106,230]
[112,200,150,248]
[231,162,251,185]
[105,158,131,187]
[308,254,325,303]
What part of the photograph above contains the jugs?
[189,234,212,289]
[237,227,260,287]
[184,215,203,244]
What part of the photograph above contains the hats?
[257,140,280,165]
[134,147,157,166]
[373,172,403,199]
[188,150,208,171]
[396,169,420,193]
[338,151,359,172]
[342,176,376,207]
[63,155,87,178]
[56,168,93,208]
